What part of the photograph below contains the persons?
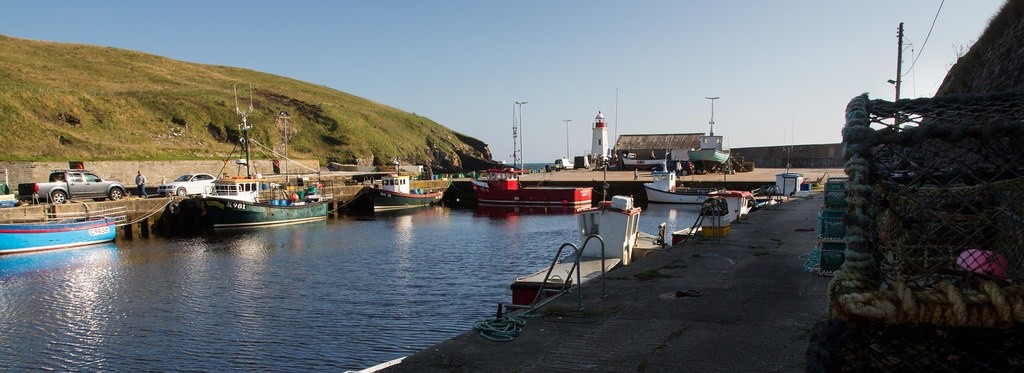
[675,159,684,178]
[634,169,638,180]
[135,170,148,199]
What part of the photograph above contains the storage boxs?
[702,224,730,238]
[702,213,730,226]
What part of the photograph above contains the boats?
[688,150,729,162]
[642,154,717,204]
[0,214,117,256]
[327,185,444,218]
[470,177,593,212]
[511,194,642,312]
[167,193,328,229]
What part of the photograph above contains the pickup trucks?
[18,168,127,204]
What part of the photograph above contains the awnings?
[688,149,730,165]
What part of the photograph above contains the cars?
[157,173,218,197]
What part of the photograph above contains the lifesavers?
[232,176,245,179]
[388,174,398,177]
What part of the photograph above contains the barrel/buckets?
[262,183,269,191]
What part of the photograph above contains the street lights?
[562,119,572,159]
[516,101,528,172]
[706,96,719,136]
